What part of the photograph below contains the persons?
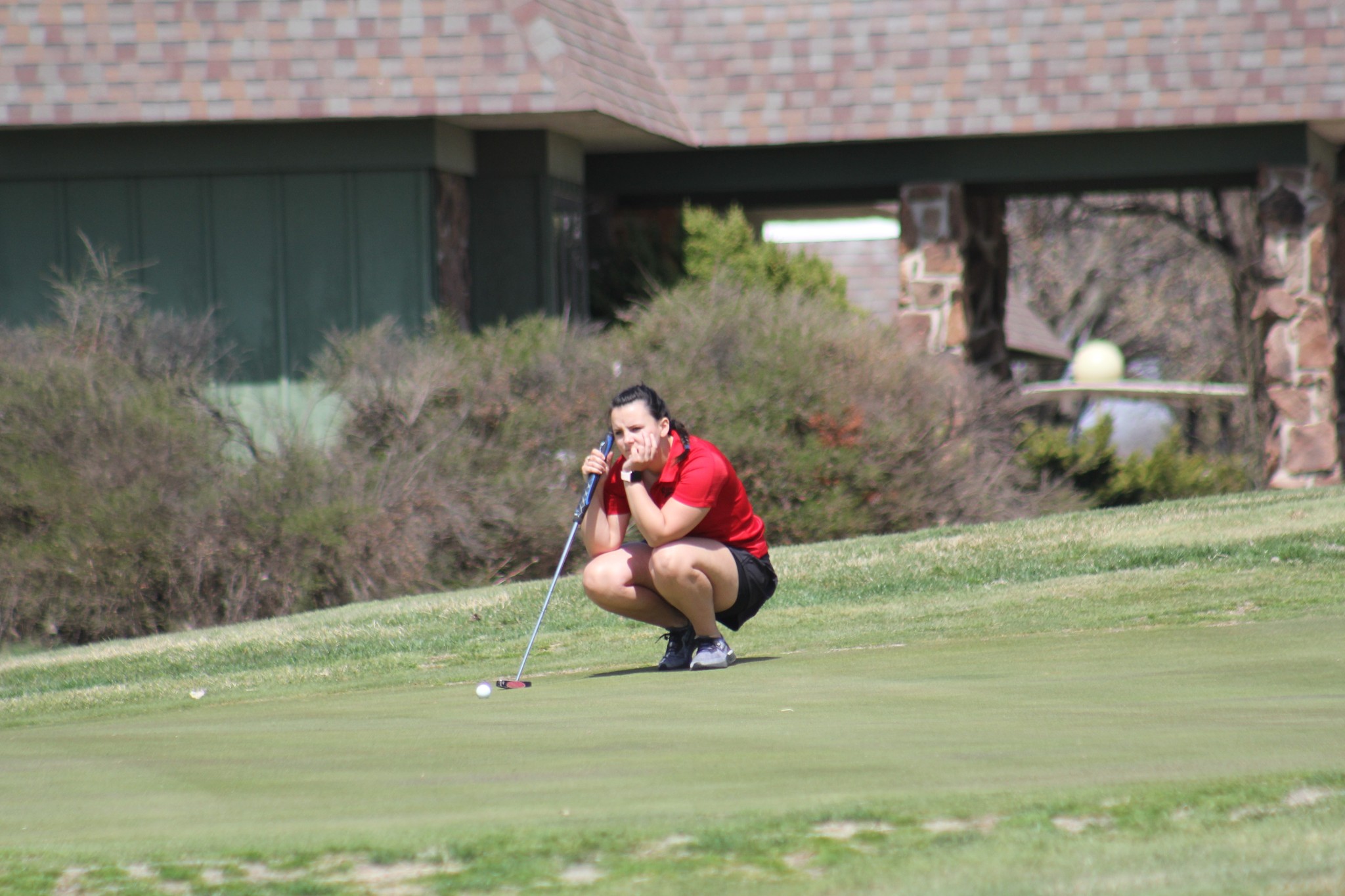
[581,383,778,672]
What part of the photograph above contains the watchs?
[620,470,642,483]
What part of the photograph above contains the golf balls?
[476,684,492,699]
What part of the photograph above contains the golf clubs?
[496,430,616,690]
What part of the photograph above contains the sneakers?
[690,635,736,670]
[655,621,695,671]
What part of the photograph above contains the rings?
[587,454,590,462]
